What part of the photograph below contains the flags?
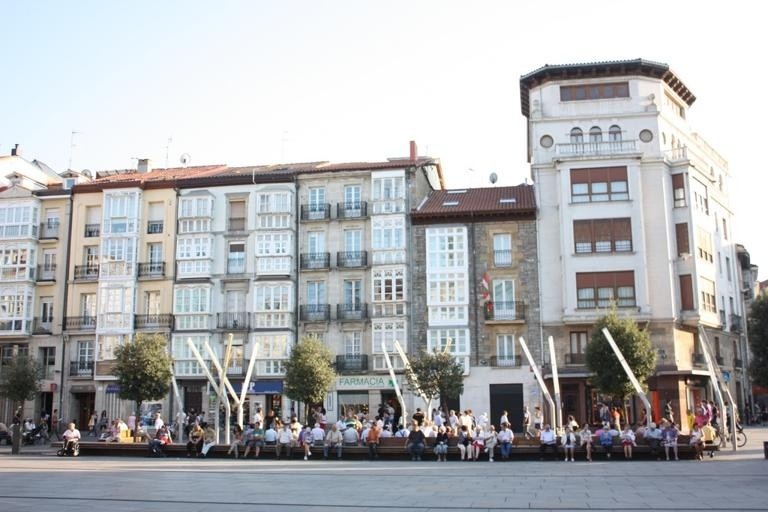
[476,270,495,320]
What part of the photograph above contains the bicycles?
[703,421,747,449]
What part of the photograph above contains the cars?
[99,416,177,442]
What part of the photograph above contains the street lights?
[739,288,754,424]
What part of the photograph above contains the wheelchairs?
[57,435,80,457]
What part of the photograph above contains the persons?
[1,399,768,458]
